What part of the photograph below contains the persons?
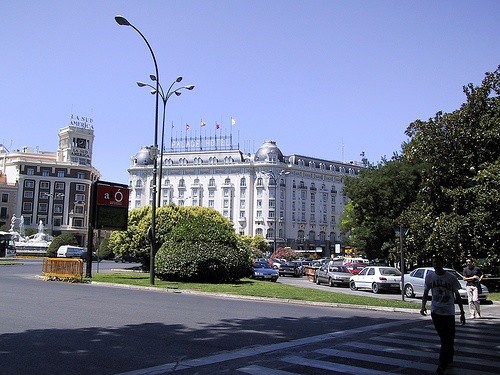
[420,255,466,375]
[462,257,484,319]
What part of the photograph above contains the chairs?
[329,269,332,271]
[333,269,337,271]
[338,268,341,271]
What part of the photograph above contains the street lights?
[260,169,291,254]
[45,192,65,235]
[113,14,160,287]
[136,73,195,207]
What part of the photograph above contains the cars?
[271,248,370,287]
[251,261,279,282]
[399,266,489,304]
[348,265,402,295]
[80,251,102,263]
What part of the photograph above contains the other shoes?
[478,313,481,317]
[472,315,475,318]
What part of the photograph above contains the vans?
[56,244,87,257]
